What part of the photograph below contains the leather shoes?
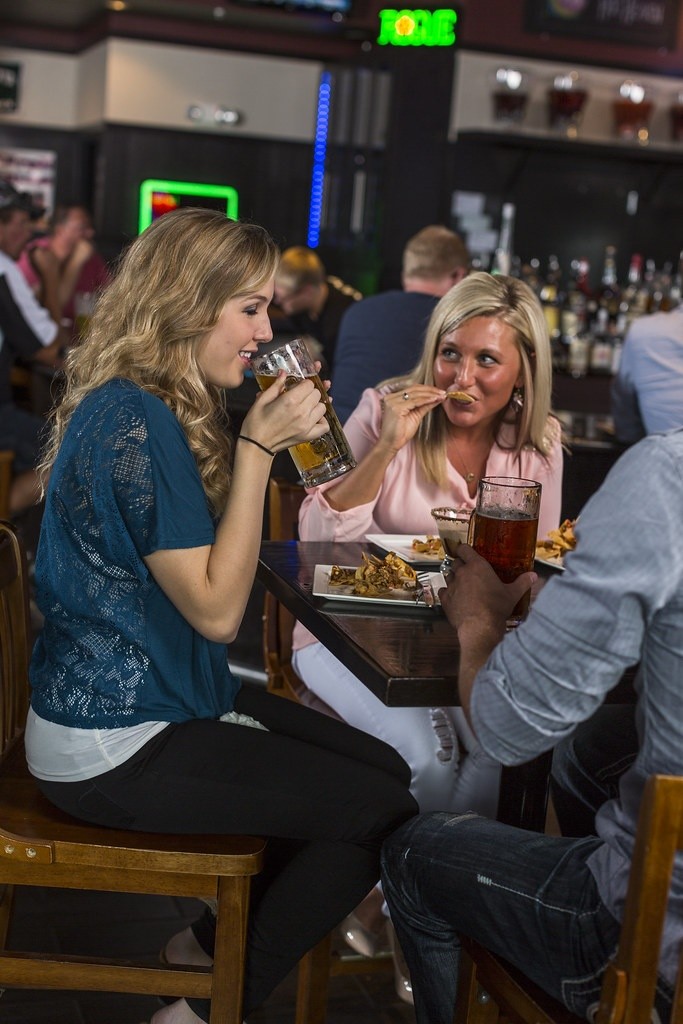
[341,911,379,958]
[389,926,415,1005]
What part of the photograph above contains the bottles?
[490,204,683,380]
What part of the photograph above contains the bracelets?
[237,434,278,458]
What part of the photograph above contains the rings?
[403,392,408,400]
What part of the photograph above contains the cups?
[75,293,92,348]
[468,475,541,629]
[488,68,683,143]
[249,338,357,490]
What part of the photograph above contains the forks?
[415,571,428,606]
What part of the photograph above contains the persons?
[271,244,356,387]
[329,224,472,429]
[610,302,683,447]
[0,181,122,557]
[381,428,683,1024]
[292,270,571,1003]
[24,209,420,1024]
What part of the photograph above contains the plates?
[312,563,449,607]
[534,555,566,570]
[364,533,444,563]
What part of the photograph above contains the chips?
[446,391,475,403]
[534,519,576,561]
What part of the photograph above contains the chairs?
[0,478,683,1024]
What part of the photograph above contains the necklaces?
[445,421,486,482]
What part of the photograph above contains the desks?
[254,542,637,833]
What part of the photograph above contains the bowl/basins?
[431,507,474,567]
